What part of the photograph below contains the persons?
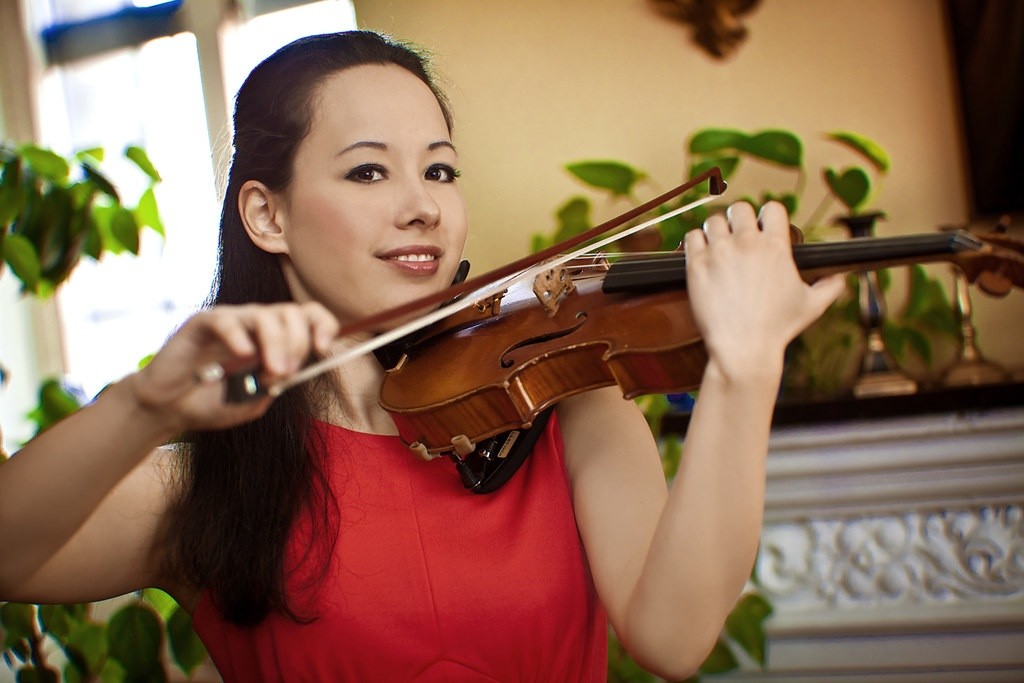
[0,32,850,683]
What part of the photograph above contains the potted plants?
[530,127,967,683]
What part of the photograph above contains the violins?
[378,209,1024,463]
